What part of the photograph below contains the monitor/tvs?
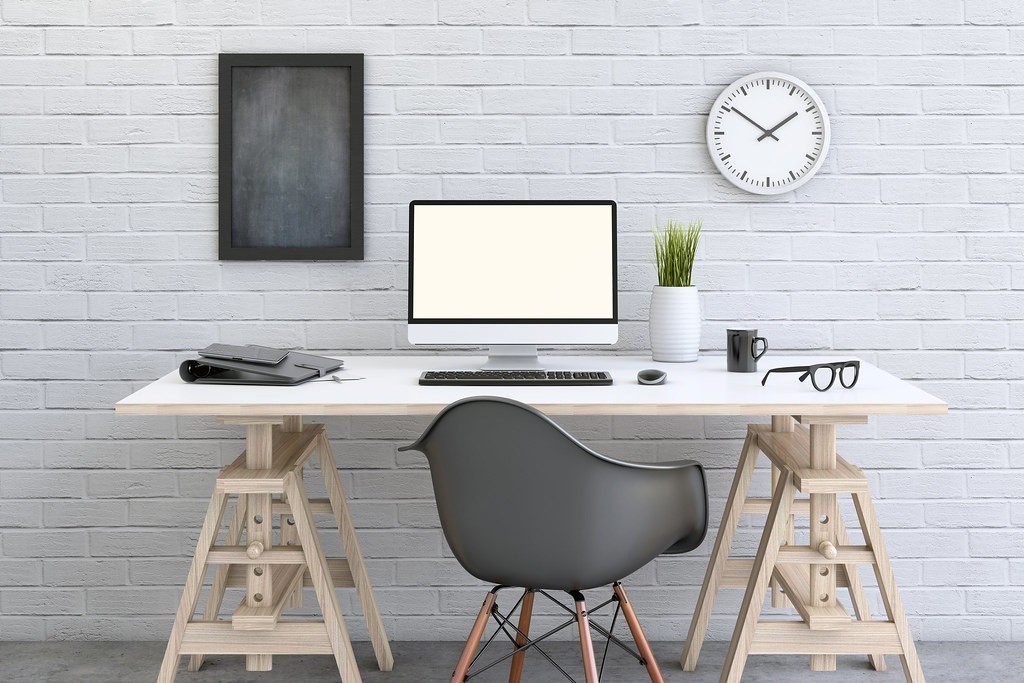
[406,199,619,371]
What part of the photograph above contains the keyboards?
[418,370,614,386]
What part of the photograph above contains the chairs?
[399,396,709,683]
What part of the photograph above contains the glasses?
[761,360,860,392]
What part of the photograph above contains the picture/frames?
[218,53,365,262]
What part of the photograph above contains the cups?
[727,328,767,373]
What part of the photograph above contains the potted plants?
[647,217,702,362]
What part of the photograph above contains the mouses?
[637,369,668,385]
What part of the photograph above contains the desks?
[114,353,949,683]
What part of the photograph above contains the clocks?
[706,71,831,195]
